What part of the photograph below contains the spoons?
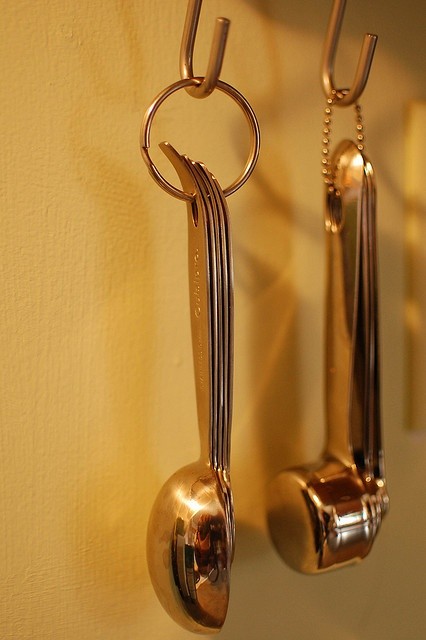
[145,140,235,636]
[266,141,391,573]
